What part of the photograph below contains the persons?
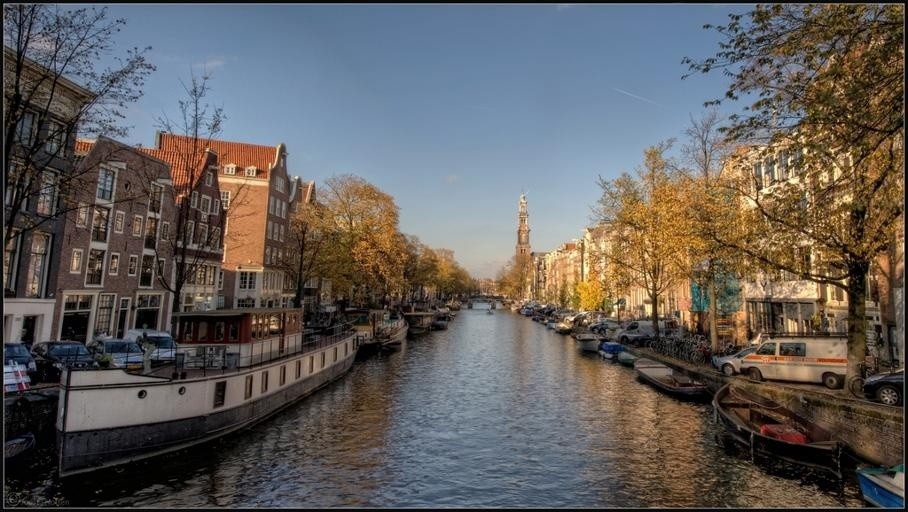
[728,344,733,353]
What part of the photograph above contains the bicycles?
[645,334,712,366]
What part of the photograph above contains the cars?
[524,299,664,343]
[4,329,176,382]
[712,336,903,406]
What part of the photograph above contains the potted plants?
[96,354,112,368]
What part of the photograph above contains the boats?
[634,358,709,395]
[599,342,623,359]
[576,333,600,352]
[351,308,456,345]
[856,465,904,508]
[56,308,359,478]
[712,383,842,482]
[618,352,638,365]
